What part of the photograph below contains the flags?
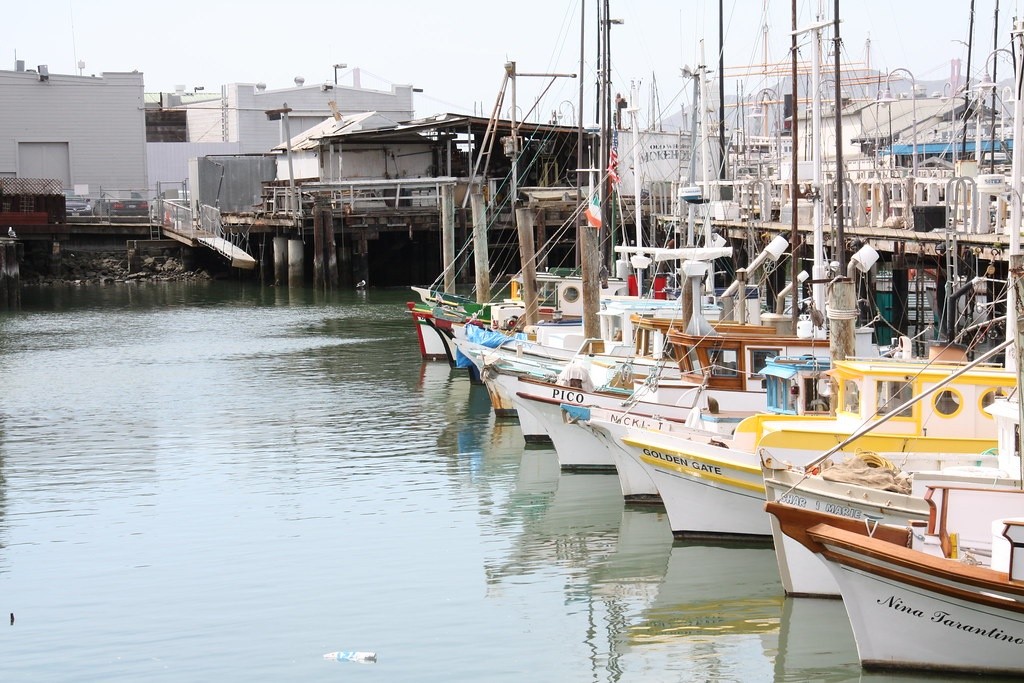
[585,194,601,229]
[609,130,619,183]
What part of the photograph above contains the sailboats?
[406,1,1024,676]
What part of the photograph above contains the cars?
[94,189,150,216]
[64,193,93,216]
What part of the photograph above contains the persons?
[665,232,679,273]
